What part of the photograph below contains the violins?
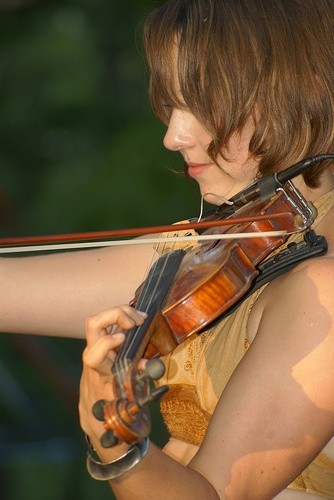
[90,176,320,449]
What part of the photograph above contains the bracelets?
[86,437,150,481]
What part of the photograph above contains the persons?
[0,1,334,500]
